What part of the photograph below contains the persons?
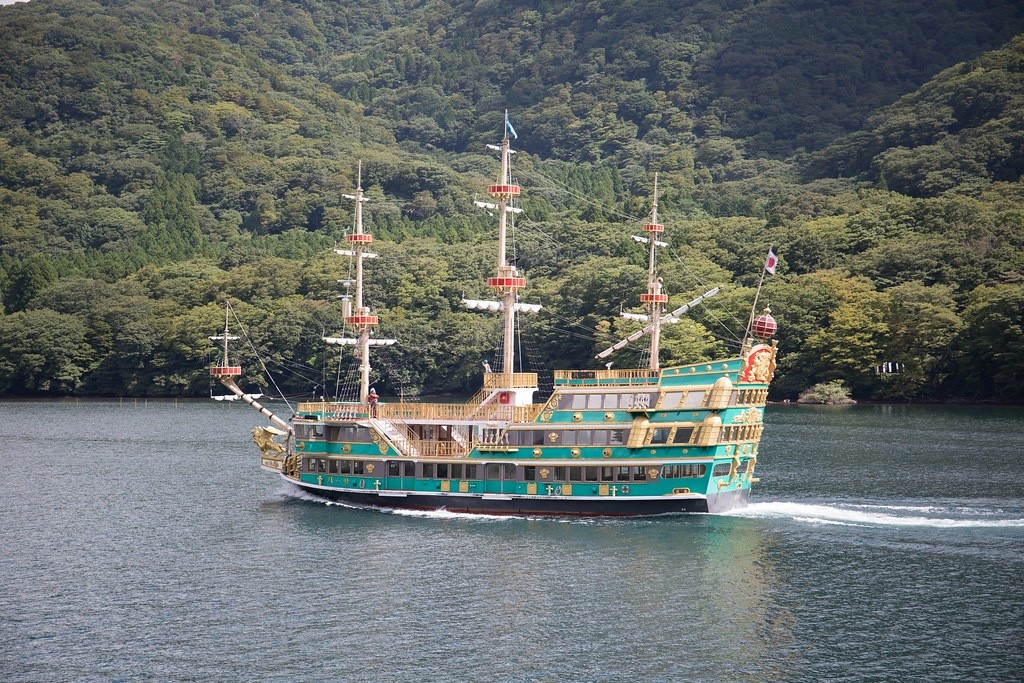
[482,360,492,373]
[370,388,379,418]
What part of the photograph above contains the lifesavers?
[621,485,630,494]
[554,486,561,496]
[359,479,366,489]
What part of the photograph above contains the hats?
[370,388,374,392]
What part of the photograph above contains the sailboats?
[208,109,778,518]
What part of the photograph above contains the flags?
[765,251,778,275]
